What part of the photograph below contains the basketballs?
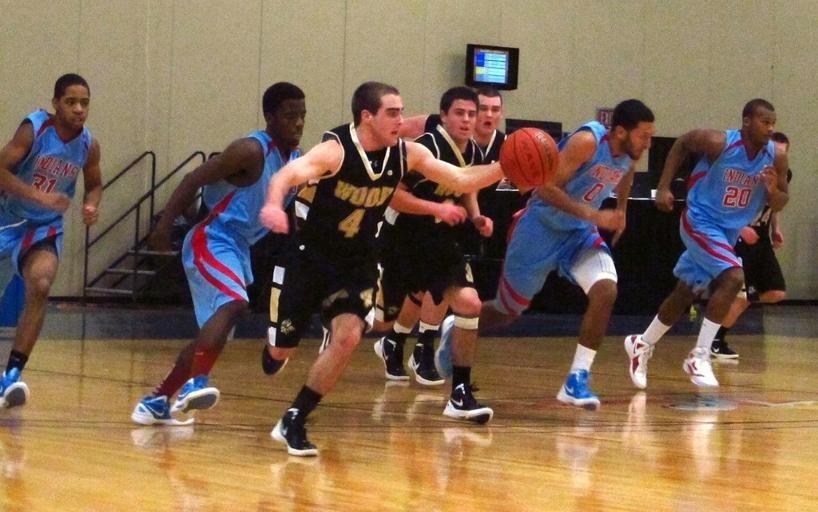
[499,127,558,188]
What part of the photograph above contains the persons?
[319,87,494,423]
[374,86,504,386]
[624,100,790,390]
[0,74,102,407]
[711,133,792,360]
[259,82,506,457]
[132,82,307,424]
[434,100,655,409]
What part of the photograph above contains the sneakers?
[0,366,31,410]
[168,374,221,423]
[433,312,456,380]
[318,325,331,355]
[555,367,602,412]
[407,341,445,386]
[440,381,494,426]
[622,331,656,390]
[709,337,741,360]
[681,346,721,388]
[130,394,196,426]
[373,332,410,381]
[260,342,296,377]
[269,406,319,457]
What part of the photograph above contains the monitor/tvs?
[648,135,702,178]
[504,117,563,144]
[464,42,520,91]
